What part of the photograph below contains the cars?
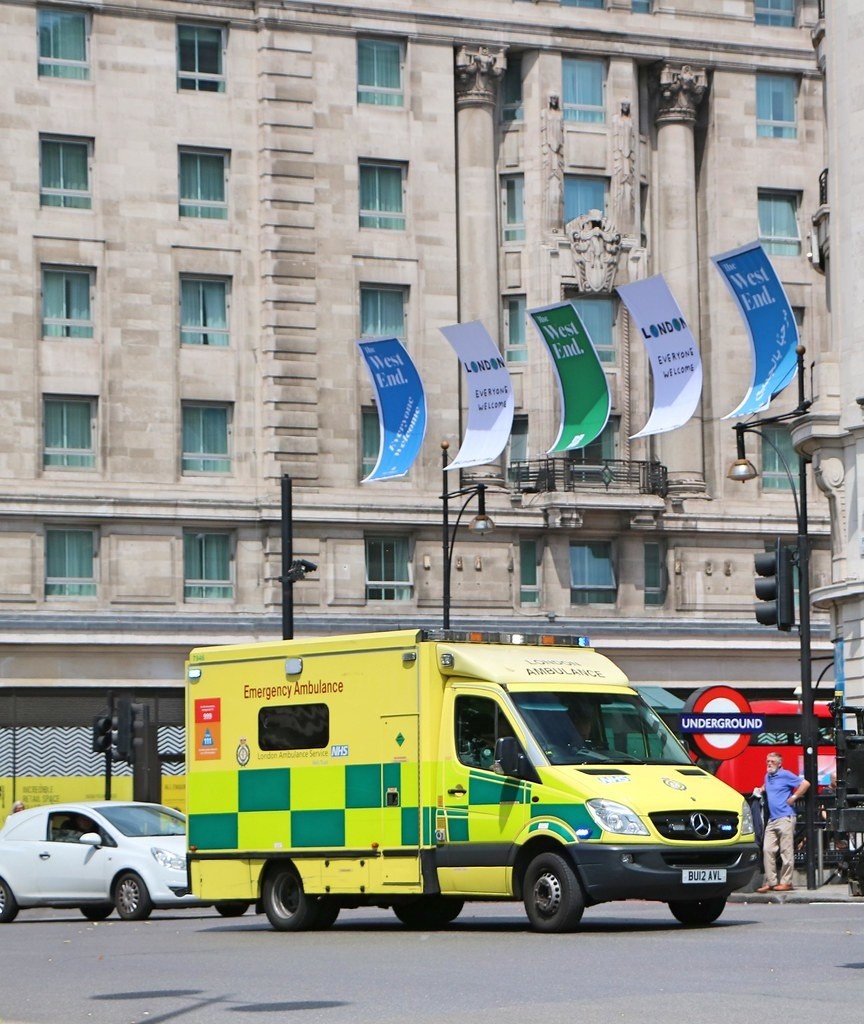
[0,800,185,924]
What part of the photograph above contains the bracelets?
[791,795,797,800]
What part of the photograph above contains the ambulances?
[185,629,758,934]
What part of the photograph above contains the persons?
[758,752,812,891]
[821,772,836,821]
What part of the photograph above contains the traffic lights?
[127,702,144,766]
[109,709,129,762]
[92,715,113,754]
[754,536,795,632]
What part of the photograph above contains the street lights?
[724,344,817,893]
[437,439,494,632]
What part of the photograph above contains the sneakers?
[758,885,793,891]
